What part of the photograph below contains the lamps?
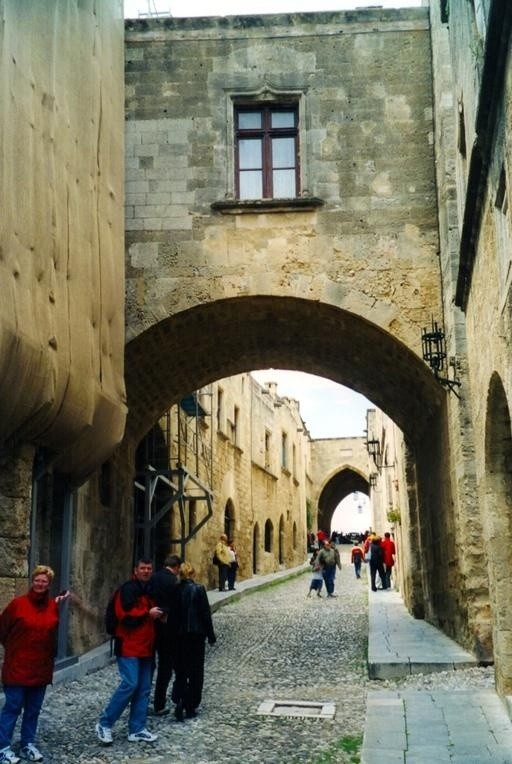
[367,437,395,471]
[369,471,378,493]
[421,314,462,399]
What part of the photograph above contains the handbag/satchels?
[213,554,221,564]
[364,551,371,561]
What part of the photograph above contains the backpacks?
[105,581,142,636]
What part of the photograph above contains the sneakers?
[1,744,22,764]
[153,704,170,716]
[96,722,114,744]
[127,729,158,743]
[19,743,43,761]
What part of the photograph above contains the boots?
[174,697,184,721]
[186,703,198,719]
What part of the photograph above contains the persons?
[95,559,168,745]
[143,553,188,712]
[226,539,238,590]
[216,534,232,592]
[307,529,395,597]
[0,565,60,764]
[167,561,216,721]
